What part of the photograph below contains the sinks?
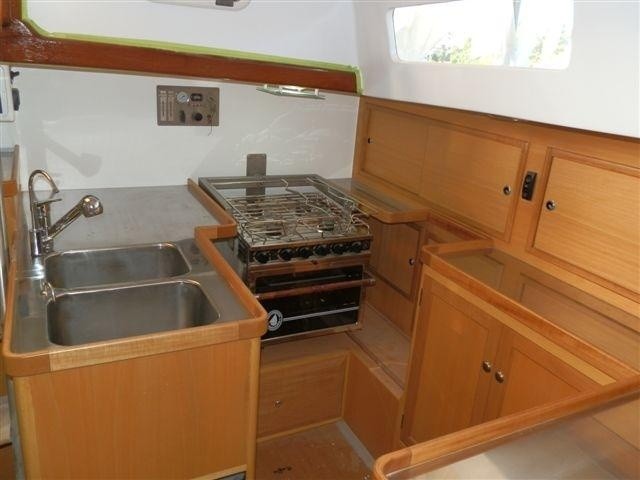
[44,241,192,289]
[45,280,220,347]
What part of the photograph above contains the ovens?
[249,252,377,346]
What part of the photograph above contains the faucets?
[33,194,104,255]
[28,169,59,257]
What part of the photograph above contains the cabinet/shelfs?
[402,266,612,450]
[366,213,424,304]
[525,145,639,304]
[359,102,533,245]
[257,356,346,444]
